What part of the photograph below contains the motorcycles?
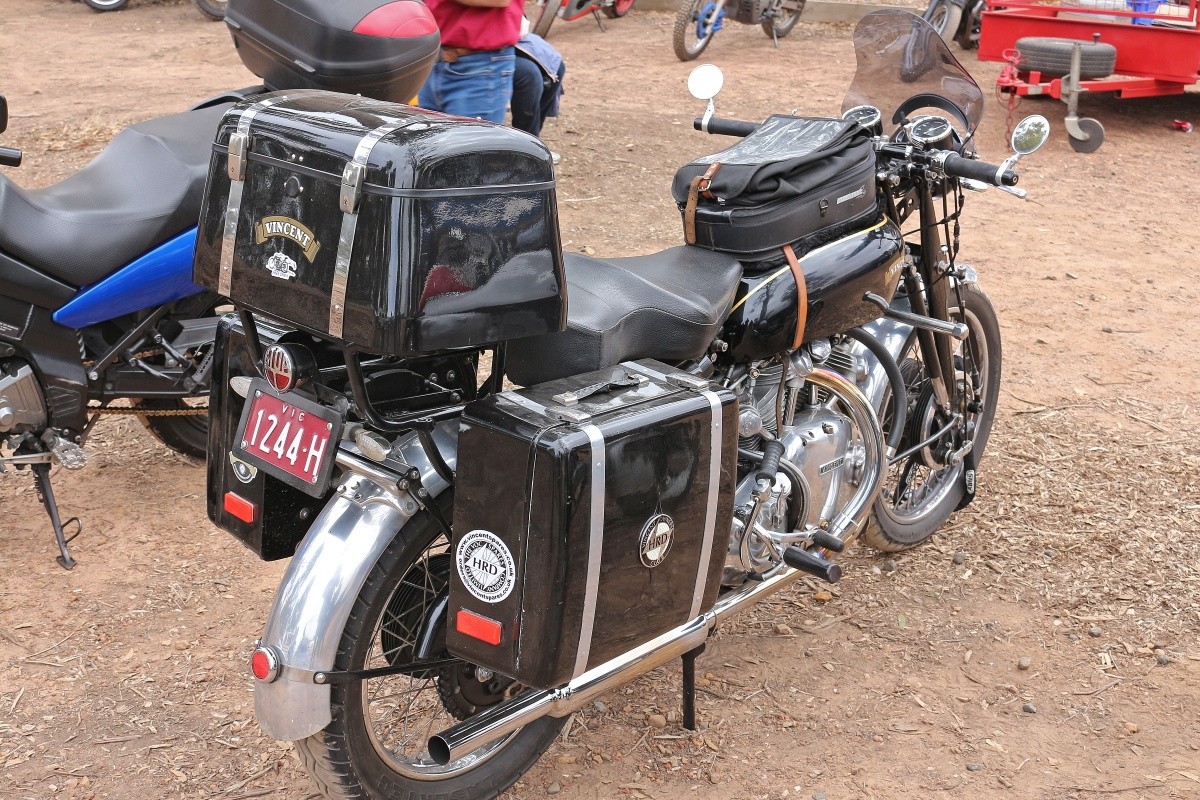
[527,0,637,40]
[675,1,807,63]
[190,7,1054,800]
[0,1,445,579]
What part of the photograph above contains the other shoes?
[550,151,560,164]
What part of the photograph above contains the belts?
[439,47,509,63]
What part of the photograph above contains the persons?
[418,0,524,123]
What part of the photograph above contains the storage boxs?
[191,87,568,358]
[446,356,738,688]
[225,0,440,104]
[208,312,478,560]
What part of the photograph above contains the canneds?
[1171,118,1192,132]
